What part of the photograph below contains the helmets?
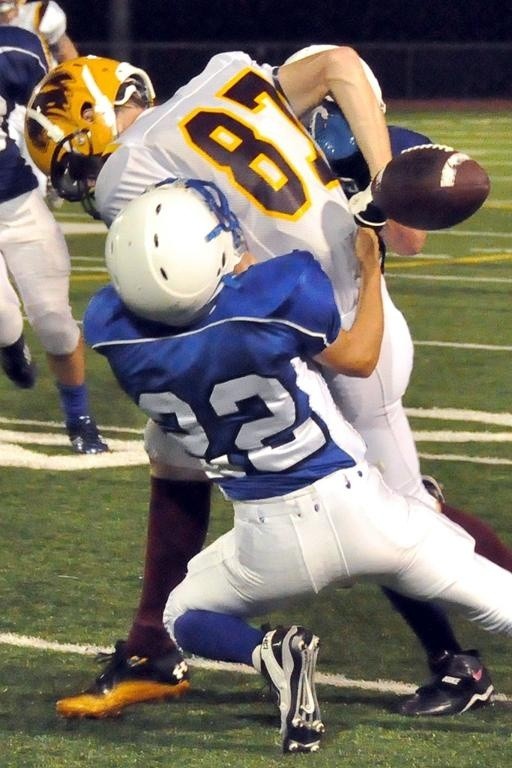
[282,44,387,197]
[24,56,157,204]
[102,177,247,330]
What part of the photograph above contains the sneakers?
[0,339,39,388]
[64,417,112,456]
[260,620,325,755]
[400,650,493,719]
[53,641,194,720]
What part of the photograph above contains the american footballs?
[371,146,489,231]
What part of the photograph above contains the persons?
[2,24,114,457]
[0,2,82,209]
[83,178,512,756]
